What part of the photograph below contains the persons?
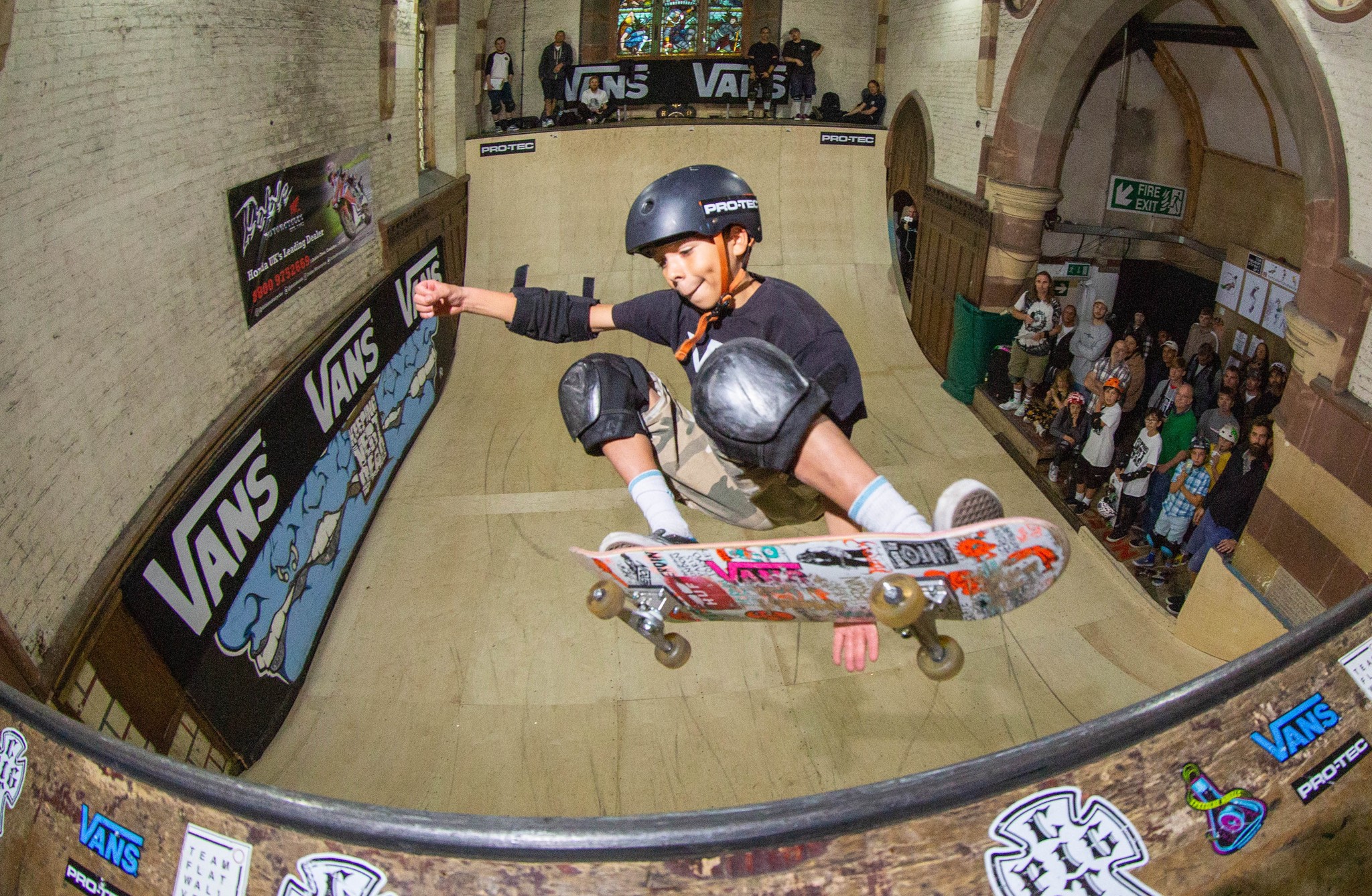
[747,26,780,120]
[997,269,1288,614]
[811,79,886,125]
[577,76,618,125]
[324,161,365,215]
[538,29,574,128]
[410,164,1007,528]
[485,37,520,133]
[896,204,918,283]
[782,28,824,121]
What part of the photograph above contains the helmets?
[1068,392,1085,404]
[1103,377,1124,393]
[1097,496,1118,518]
[1219,424,1240,443]
[1269,362,1287,374]
[626,165,762,255]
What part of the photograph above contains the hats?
[1160,340,1177,350]
[1095,298,1109,310]
[1189,435,1211,449]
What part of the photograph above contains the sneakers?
[599,528,700,552]
[802,114,810,121]
[794,113,802,121]
[763,111,774,120]
[506,124,519,131]
[586,117,598,124]
[1000,399,1029,416]
[495,126,502,132]
[933,478,1004,533]
[1106,529,1192,614]
[1049,459,1092,514]
[746,110,754,120]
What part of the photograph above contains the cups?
[1209,450,1220,466]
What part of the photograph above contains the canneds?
[617,109,620,116]
[618,116,620,121]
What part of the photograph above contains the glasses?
[1144,417,1159,423]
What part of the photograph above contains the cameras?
[904,217,913,222]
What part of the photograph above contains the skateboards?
[496,115,540,131]
[1102,471,1124,529]
[568,517,1070,681]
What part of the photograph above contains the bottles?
[558,107,564,119]
[617,107,621,122]
